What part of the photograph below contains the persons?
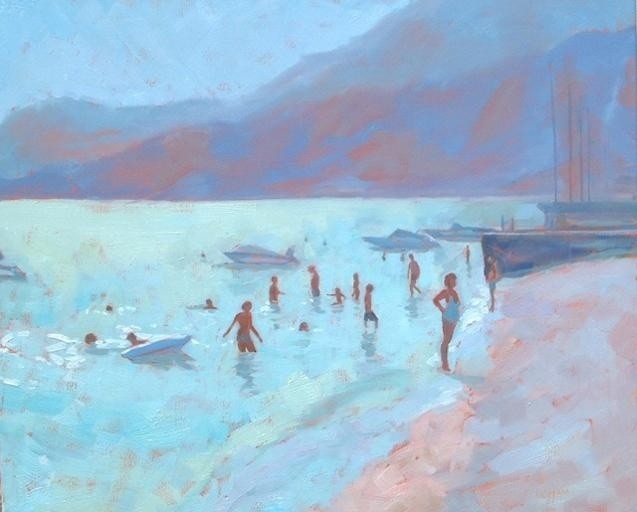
[234,352,258,393]
[305,265,320,297]
[326,288,345,306]
[406,254,421,298]
[432,272,461,372]
[350,271,360,299]
[464,244,470,264]
[360,331,379,360]
[404,296,417,322]
[363,283,378,329]
[203,299,217,310]
[299,322,311,332]
[268,276,284,303]
[222,300,263,352]
[84,333,98,345]
[484,255,498,311]
[124,332,148,348]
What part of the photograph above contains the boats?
[120,334,191,360]
[360,228,442,250]
[0,263,27,279]
[223,242,299,266]
[419,223,503,237]
